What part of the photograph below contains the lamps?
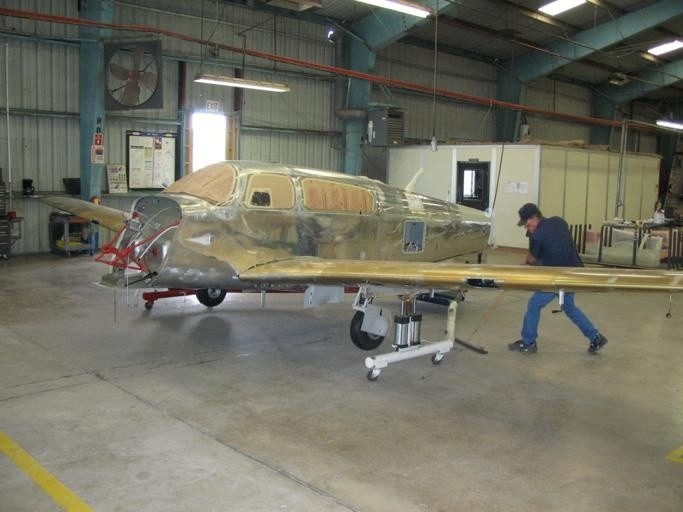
[193,1,290,93]
[358,1,436,20]
[607,53,631,87]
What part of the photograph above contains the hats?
[516,202,538,227]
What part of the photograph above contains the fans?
[104,39,163,111]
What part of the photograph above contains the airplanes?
[39,158,681,352]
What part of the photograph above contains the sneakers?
[586,333,609,353]
[506,338,538,354]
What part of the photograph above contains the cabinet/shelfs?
[46,209,94,259]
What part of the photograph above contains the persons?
[507,203,607,354]
[652,199,665,223]
